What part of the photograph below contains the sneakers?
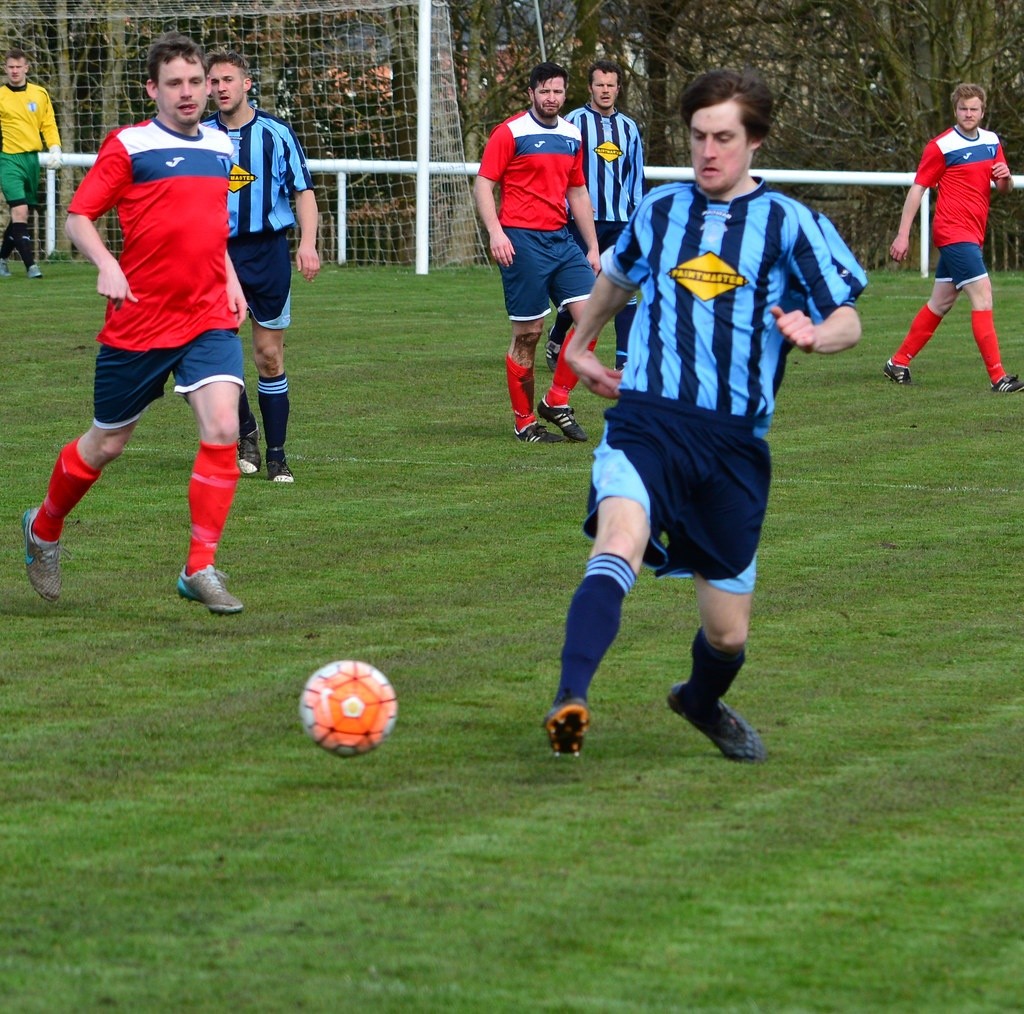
[545,689,589,755]
[667,681,766,762]
[0,258,12,276]
[537,394,588,442]
[27,265,43,278]
[514,422,565,443]
[990,375,1024,392]
[266,458,294,483]
[544,325,566,373]
[20,508,73,602]
[883,358,918,385]
[236,413,262,474]
[177,564,243,616]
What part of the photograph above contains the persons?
[196,45,320,484]
[883,84,1024,395]
[20,31,252,614]
[544,73,867,759]
[474,60,602,446]
[0,52,63,281]
[542,63,643,376]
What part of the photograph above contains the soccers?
[298,659,399,758]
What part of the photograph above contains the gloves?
[47,144,64,170]
